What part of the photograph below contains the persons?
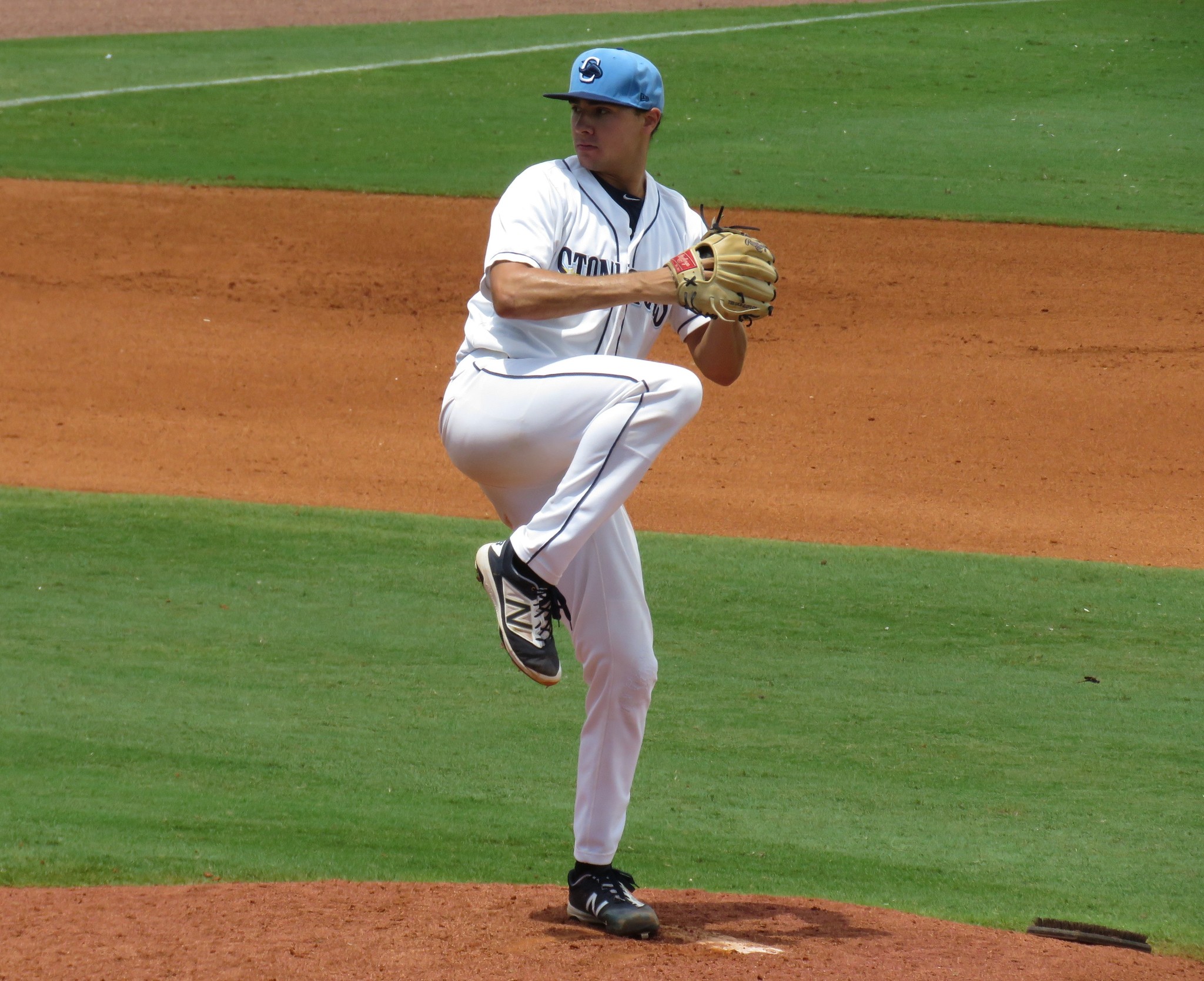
[438,48,779,940]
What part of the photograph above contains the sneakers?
[567,866,660,939]
[474,539,574,685]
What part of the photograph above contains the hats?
[542,48,665,113]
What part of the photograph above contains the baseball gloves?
[662,231,780,323]
